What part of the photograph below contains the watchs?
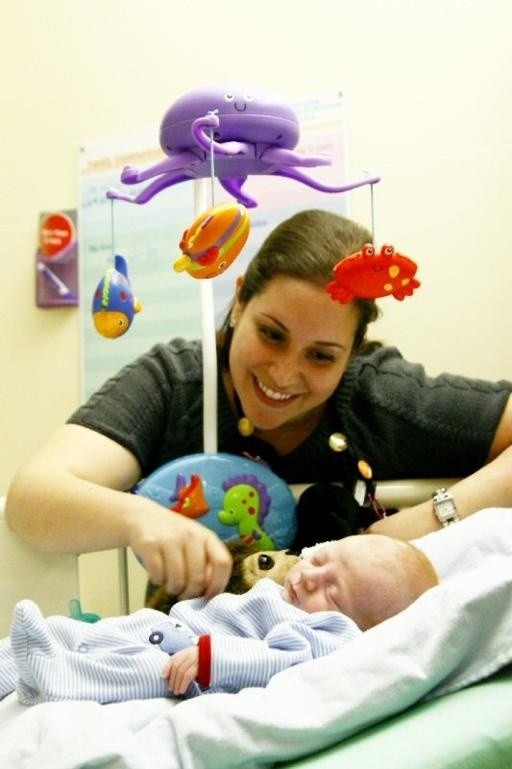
[431,487,460,529]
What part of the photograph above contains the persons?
[0,533,438,706]
[4,208,512,600]
